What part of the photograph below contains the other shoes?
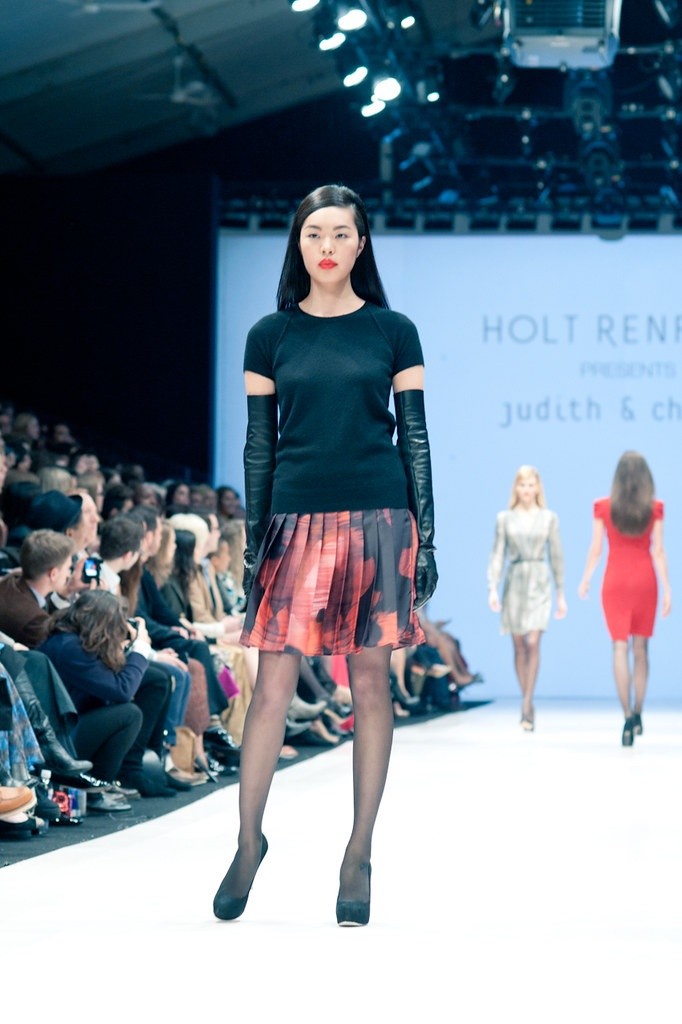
[34,787,61,819]
[117,769,177,797]
[48,807,81,826]
[162,772,191,791]
[198,664,477,780]
[50,770,105,791]
[165,767,206,786]
[98,778,140,801]
[0,787,33,813]
[86,787,132,812]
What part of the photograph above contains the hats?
[28,490,82,531]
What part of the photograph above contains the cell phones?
[81,558,100,587]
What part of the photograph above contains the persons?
[212,184,438,927]
[581,452,671,746]
[0,412,484,845]
[489,466,567,731]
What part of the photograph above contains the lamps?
[290,0,441,117]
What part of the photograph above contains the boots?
[15,667,92,775]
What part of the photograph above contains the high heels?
[632,712,642,735]
[521,712,534,732]
[213,833,269,920]
[336,862,373,926]
[623,718,633,747]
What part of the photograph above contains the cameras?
[124,618,137,639]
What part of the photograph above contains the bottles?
[40,769,54,834]
